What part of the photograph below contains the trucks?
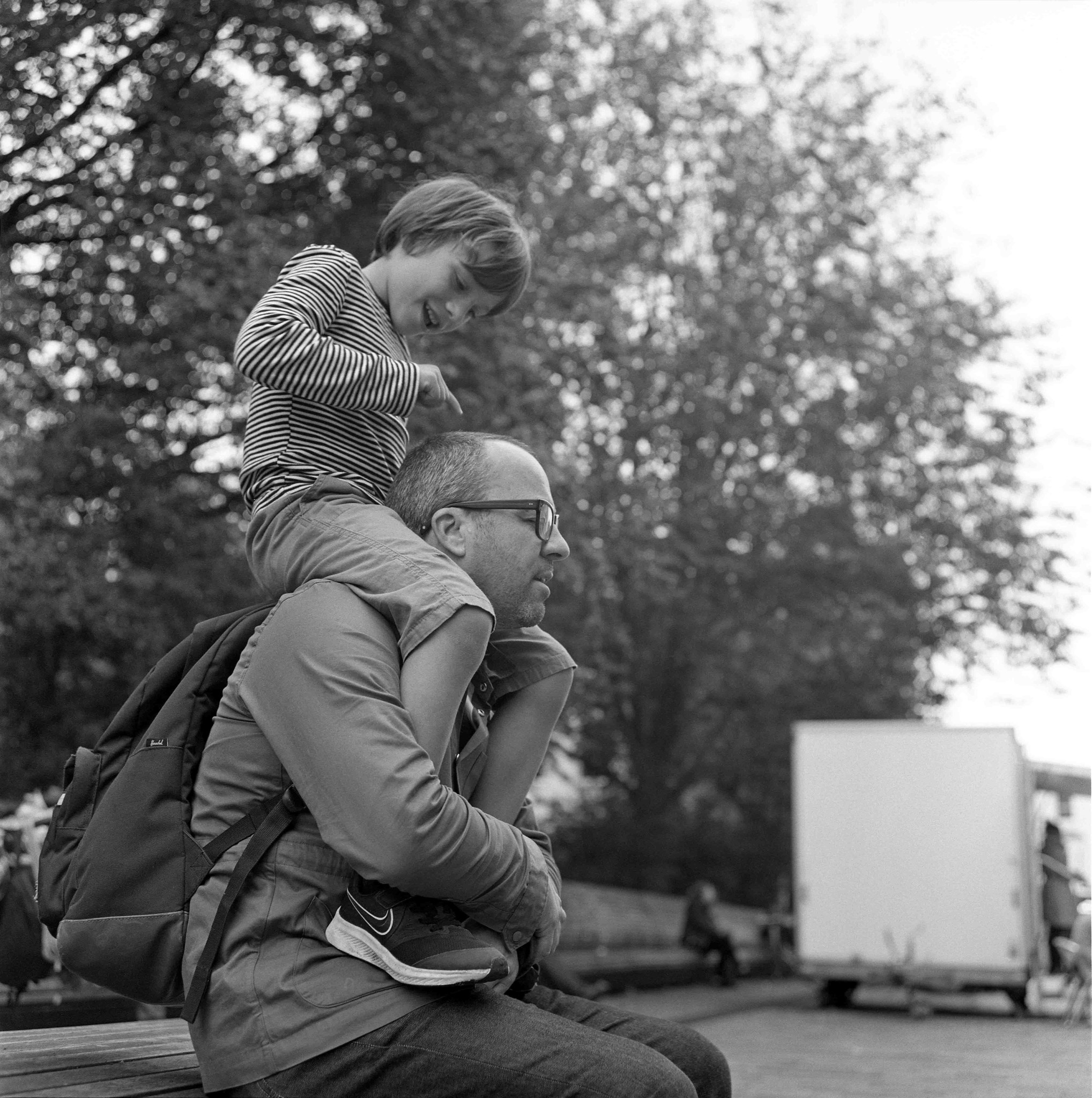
[789,718,1092,1004]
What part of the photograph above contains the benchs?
[0,1019,203,1098]
[550,884,767,983]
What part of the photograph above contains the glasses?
[418,498,561,542]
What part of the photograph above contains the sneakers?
[324,873,512,988]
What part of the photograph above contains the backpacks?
[33,601,318,1023]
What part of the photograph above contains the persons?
[1041,823,1078,976]
[233,175,580,988]
[181,430,732,1098]
[683,884,736,986]
[0,836,56,1009]
